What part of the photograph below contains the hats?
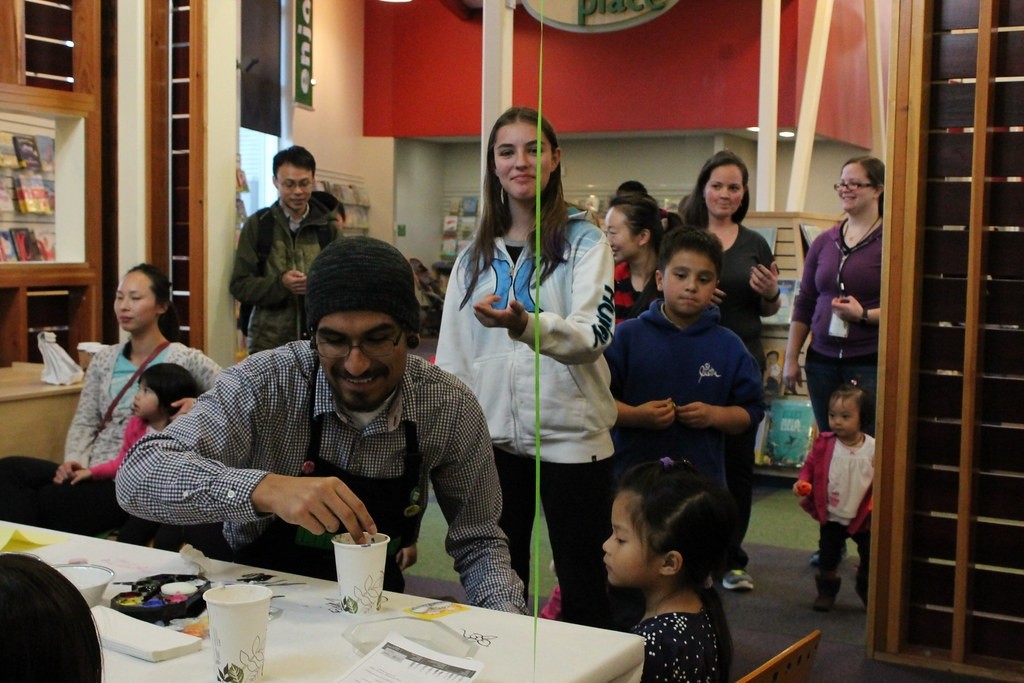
[304,236,420,330]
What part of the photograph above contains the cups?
[330,531,391,616]
[201,584,273,683]
[77,342,109,371]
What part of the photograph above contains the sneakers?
[722,570,753,589]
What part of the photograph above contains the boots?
[813,571,841,610]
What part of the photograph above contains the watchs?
[861,309,868,326]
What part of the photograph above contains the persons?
[114,235,528,616]
[0,264,225,559]
[539,150,885,683]
[228,145,348,356]
[433,107,619,631]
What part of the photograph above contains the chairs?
[0,453,59,519]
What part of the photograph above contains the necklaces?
[838,434,863,446]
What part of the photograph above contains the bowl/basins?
[53,564,115,609]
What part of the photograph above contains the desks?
[0,361,88,467]
[0,519,644,683]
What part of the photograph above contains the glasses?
[834,183,874,193]
[275,177,314,190]
[303,325,404,359]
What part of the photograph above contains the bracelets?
[763,288,781,303]
